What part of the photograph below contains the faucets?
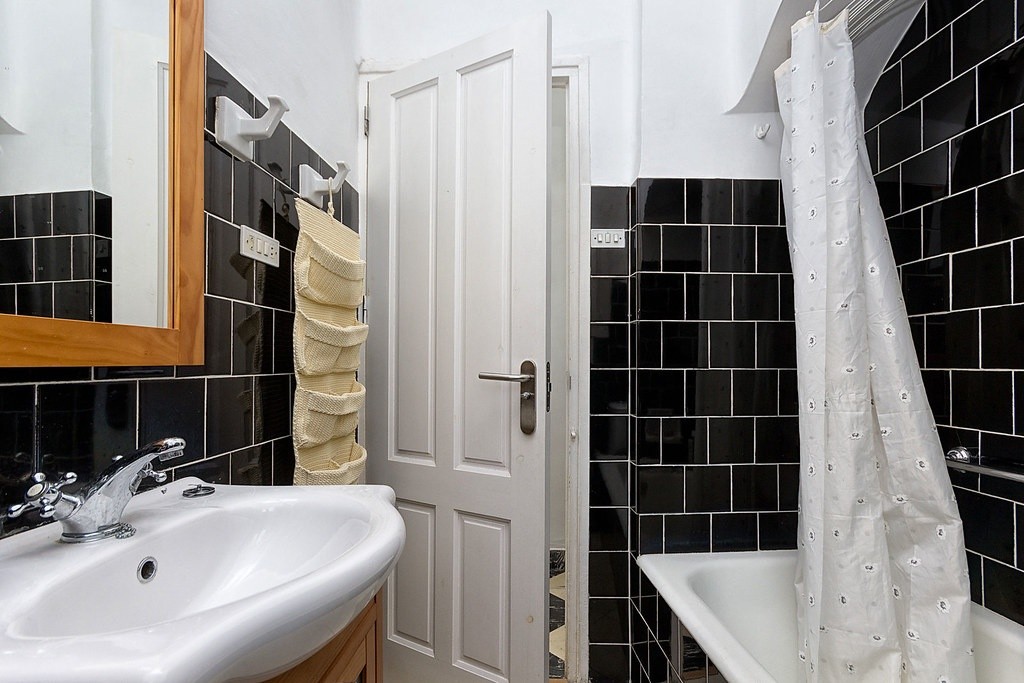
[7,435,187,544]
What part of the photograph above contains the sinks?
[1,476,405,683]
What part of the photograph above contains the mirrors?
[0,0,206,368]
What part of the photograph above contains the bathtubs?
[637,551,1024,683]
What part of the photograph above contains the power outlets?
[240,225,280,268]
